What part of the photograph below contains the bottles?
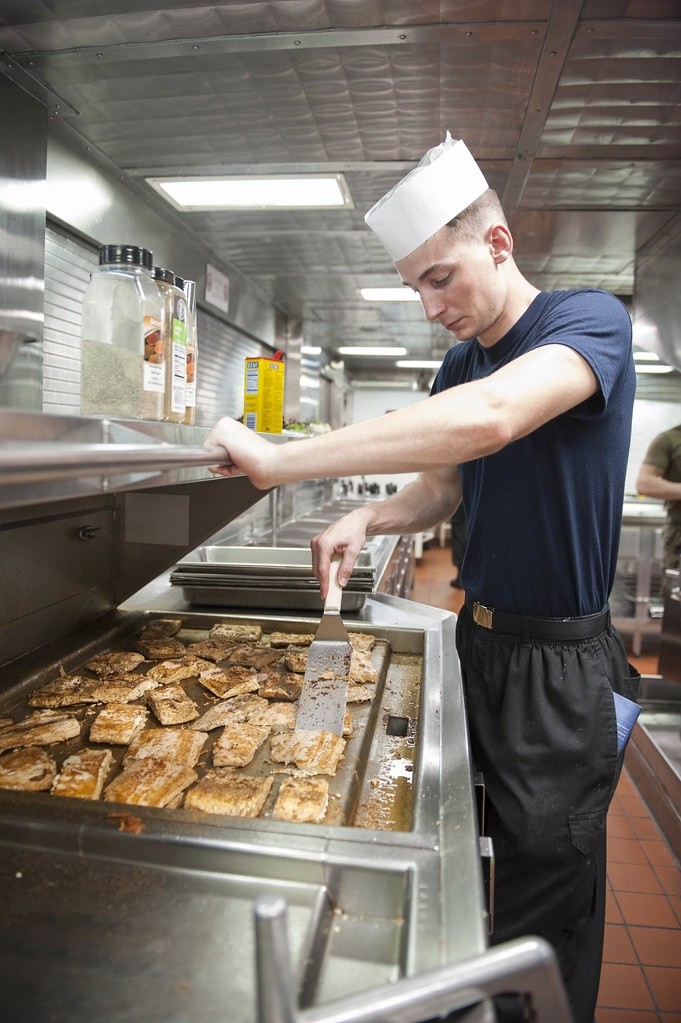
[82,243,198,426]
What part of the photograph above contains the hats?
[363,131,489,262]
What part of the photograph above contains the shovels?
[293,555,353,741]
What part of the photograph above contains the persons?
[207,139,644,1022]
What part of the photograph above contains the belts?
[462,592,611,641]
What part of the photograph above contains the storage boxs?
[244,355,286,434]
